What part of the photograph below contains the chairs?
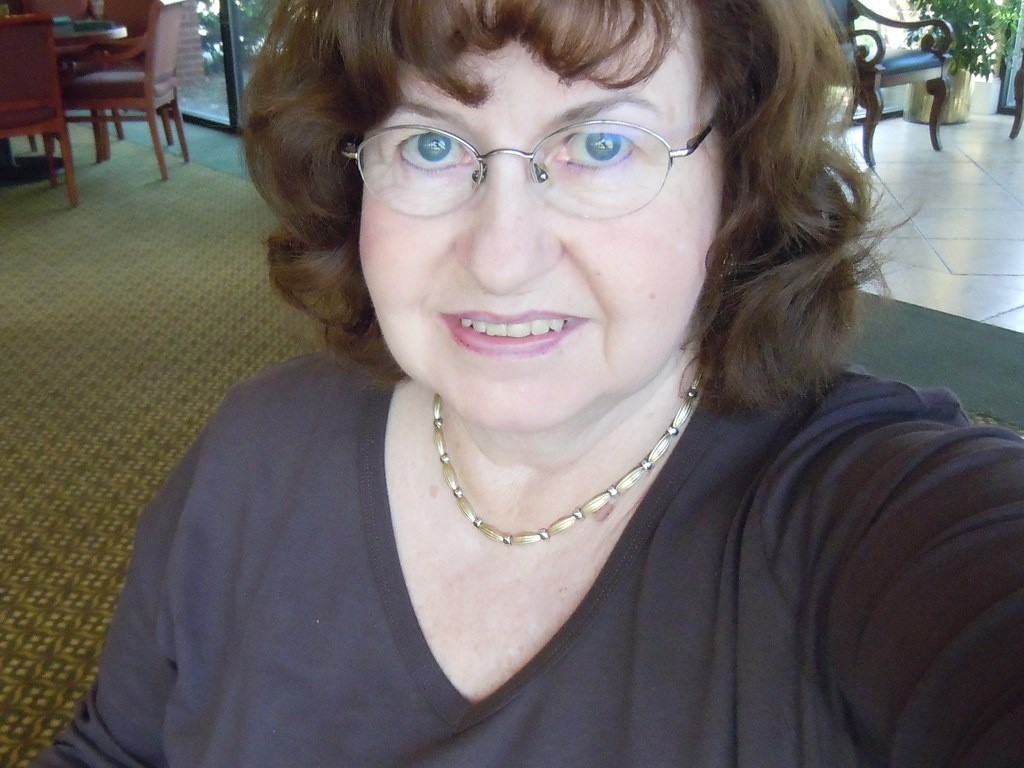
[0,0,189,207]
[821,0,953,167]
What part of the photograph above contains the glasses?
[342,98,724,217]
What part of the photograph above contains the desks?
[53,19,128,161]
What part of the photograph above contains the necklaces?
[432,363,703,543]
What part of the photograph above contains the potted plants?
[903,0,1024,124]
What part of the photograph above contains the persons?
[24,0,1024,768]
